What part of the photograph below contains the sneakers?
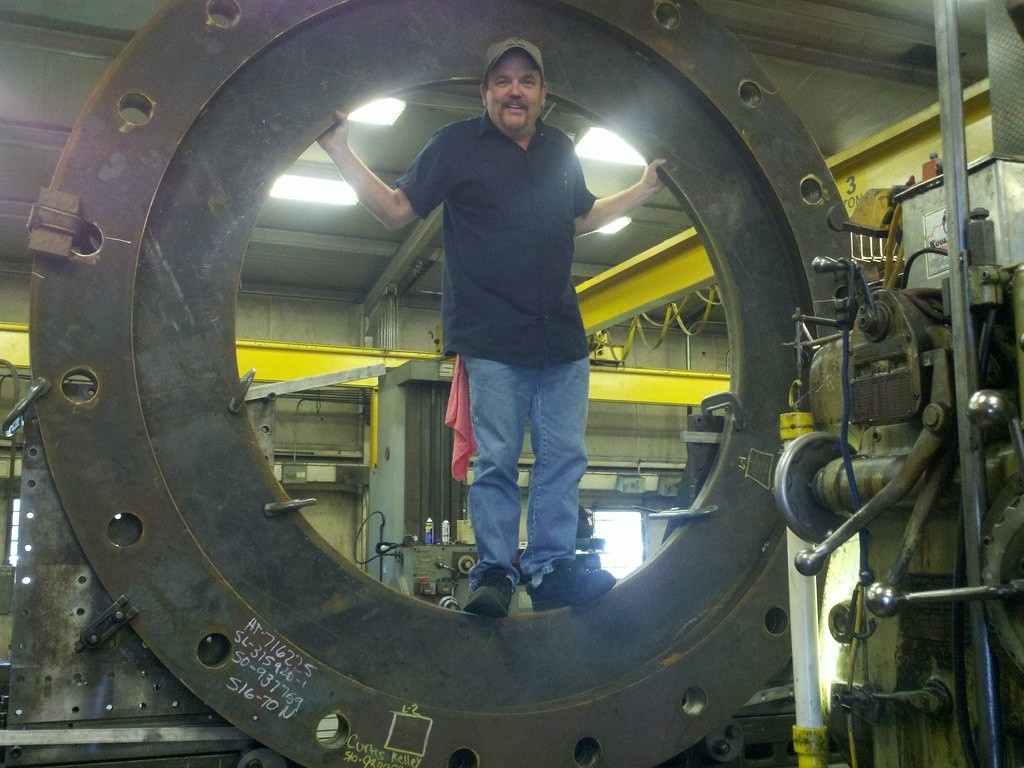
[527,557,614,610]
[462,568,513,615]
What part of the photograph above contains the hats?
[483,37,545,82]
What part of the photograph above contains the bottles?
[442,520,450,545]
[426,518,435,545]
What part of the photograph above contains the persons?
[312,35,670,621]
